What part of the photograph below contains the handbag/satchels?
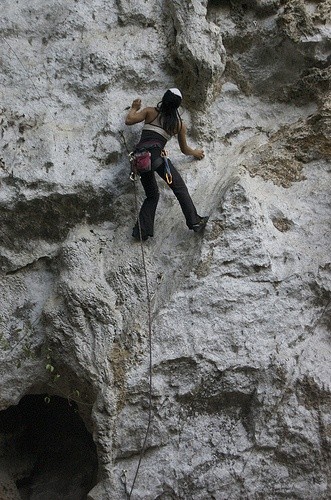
[133,149,152,173]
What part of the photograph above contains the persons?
[124,87,210,242]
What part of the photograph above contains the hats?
[162,87,183,107]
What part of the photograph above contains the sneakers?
[192,216,209,232]
[132,226,148,240]
